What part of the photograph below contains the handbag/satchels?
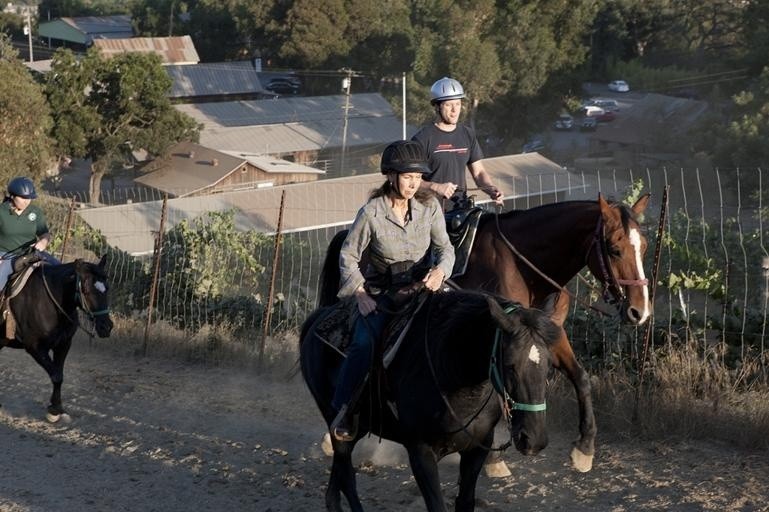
[369,252,430,289]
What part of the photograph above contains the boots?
[335,342,373,441]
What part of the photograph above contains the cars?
[606,79,631,93]
[553,98,622,134]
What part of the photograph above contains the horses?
[296,287,564,511]
[286,190,654,481]
[0,252,117,424]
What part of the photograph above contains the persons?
[327,136,455,450]
[0,175,63,293]
[409,75,504,226]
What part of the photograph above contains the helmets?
[7,176,38,199]
[380,139,433,176]
[429,76,467,107]
